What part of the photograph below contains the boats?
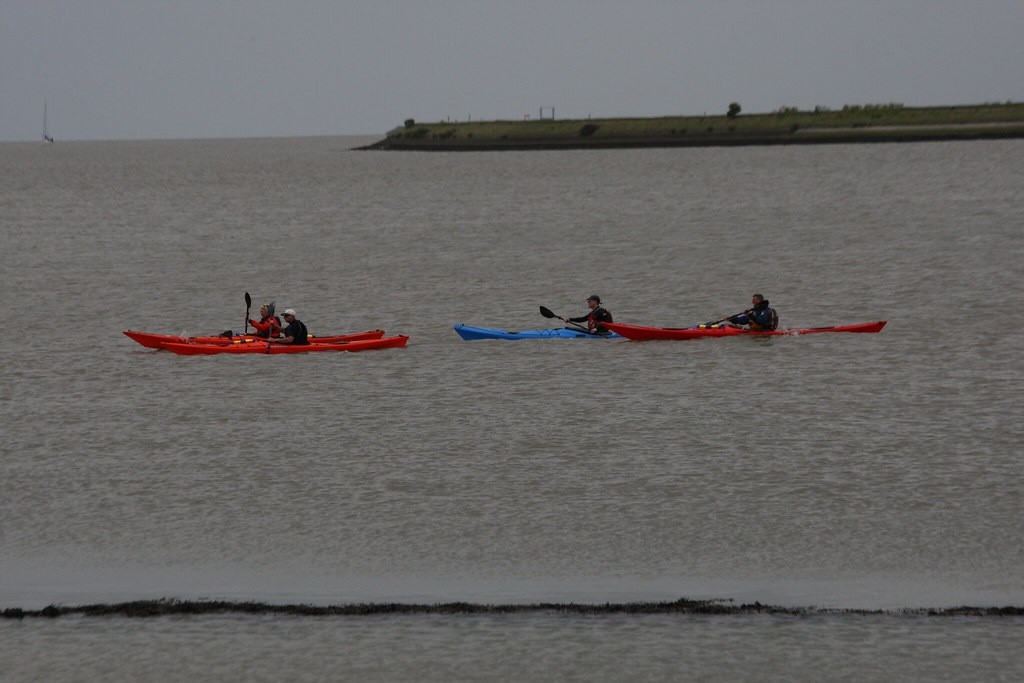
[596,319,889,340]
[454,323,619,340]
[121,327,410,354]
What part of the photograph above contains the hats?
[586,295,603,304]
[280,308,297,317]
[261,303,270,310]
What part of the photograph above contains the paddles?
[704,300,769,325]
[540,306,610,340]
[244,291,277,355]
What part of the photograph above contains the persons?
[268,309,308,345]
[564,296,613,334]
[724,294,772,331]
[245,303,281,338]
